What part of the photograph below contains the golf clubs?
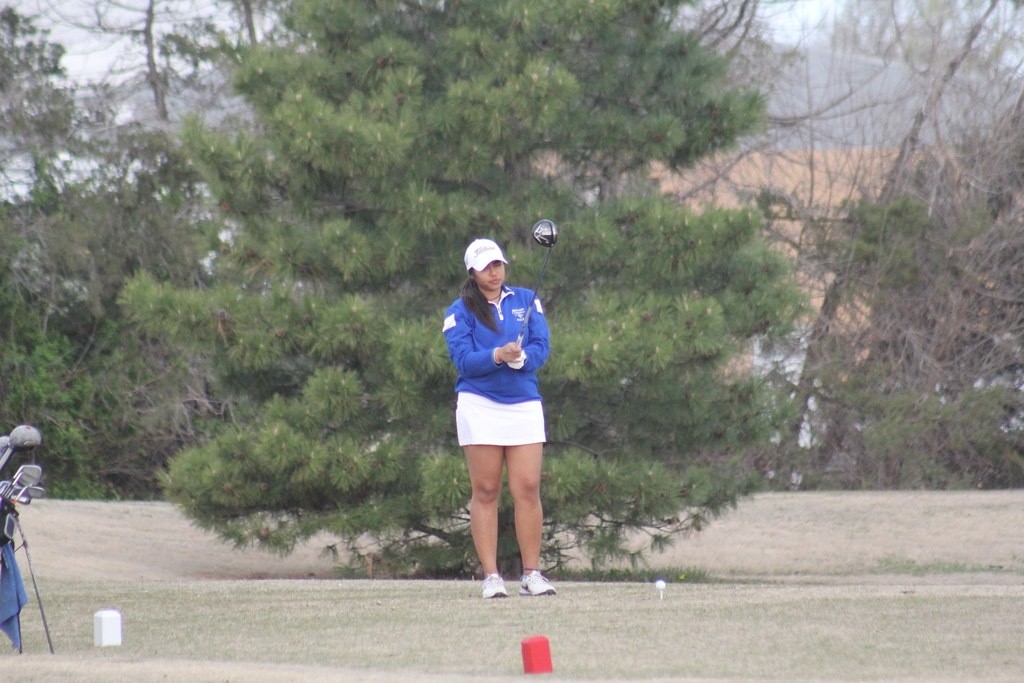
[0,465,43,500]
[0,424,42,471]
[9,483,45,505]
[515,219,560,348]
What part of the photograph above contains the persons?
[443,238,556,597]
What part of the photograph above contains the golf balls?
[655,580,666,590]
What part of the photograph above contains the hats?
[464,238,509,272]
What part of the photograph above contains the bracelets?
[492,347,503,364]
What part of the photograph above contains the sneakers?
[481,573,509,598]
[518,570,557,596]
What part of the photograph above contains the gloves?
[507,350,526,370]
